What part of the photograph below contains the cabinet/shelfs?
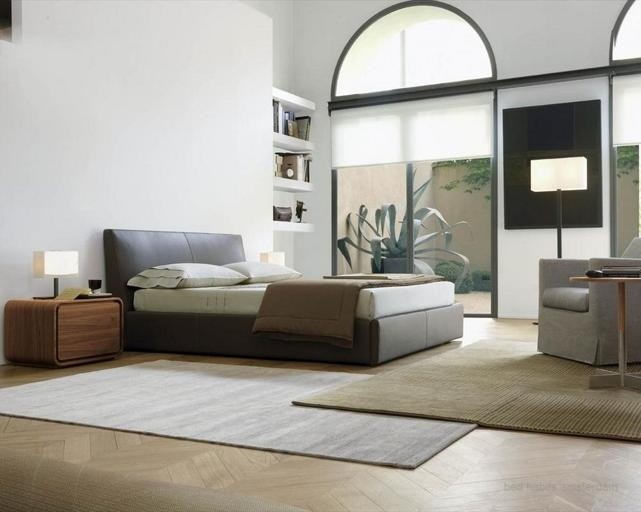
[271,87,316,235]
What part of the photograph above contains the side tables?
[570,274,641,391]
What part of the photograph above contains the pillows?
[222,262,304,285]
[126,262,250,289]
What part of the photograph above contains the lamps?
[259,251,286,265]
[528,154,588,258]
[32,250,79,299]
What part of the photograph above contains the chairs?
[535,237,641,366]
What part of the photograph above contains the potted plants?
[336,167,469,290]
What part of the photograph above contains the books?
[54,285,92,301]
[273,98,311,184]
[602,271,640,275]
[601,275,641,278]
[602,265,641,271]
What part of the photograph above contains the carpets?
[0,358,479,470]
[291,336,641,442]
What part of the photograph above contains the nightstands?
[4,296,125,369]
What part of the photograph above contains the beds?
[103,231,463,367]
